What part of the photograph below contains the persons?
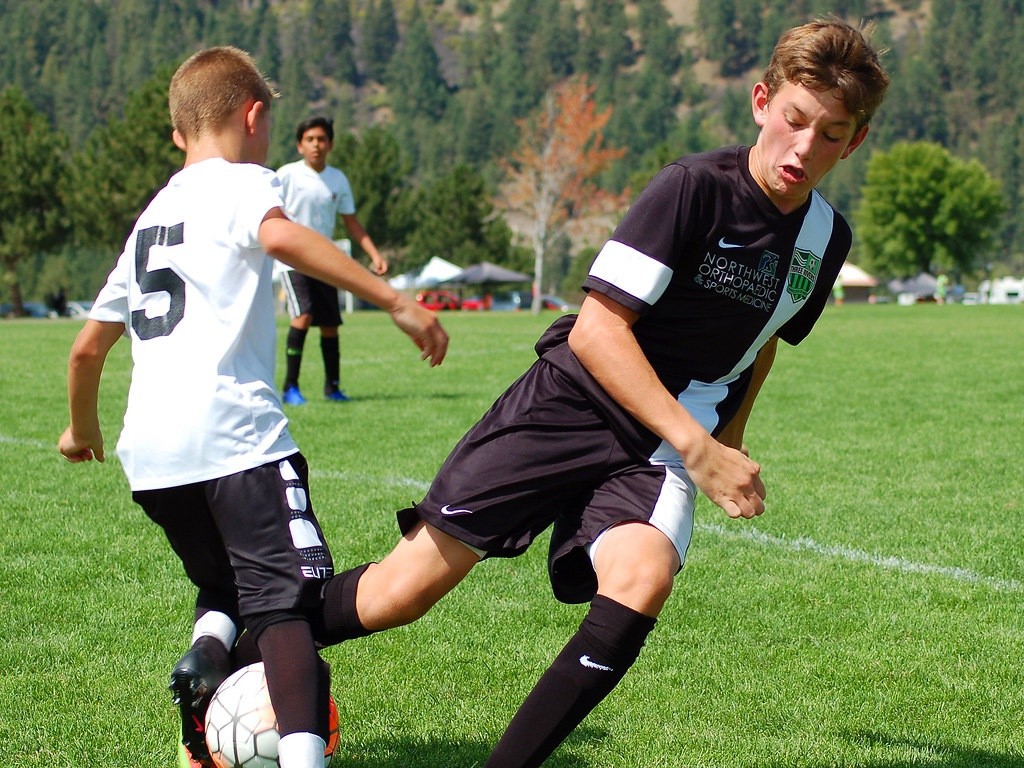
[934,270,951,305]
[832,275,842,304]
[178,18,887,768]
[272,118,388,404]
[56,45,448,768]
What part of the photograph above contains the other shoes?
[328,391,346,400]
[283,386,305,404]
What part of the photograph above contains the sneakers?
[168,651,219,768]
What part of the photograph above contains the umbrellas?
[389,258,528,312]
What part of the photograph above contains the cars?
[491,291,572,311]
[416,285,493,312]
[0,303,53,318]
[65,301,94,315]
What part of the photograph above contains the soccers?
[204,661,341,768]
